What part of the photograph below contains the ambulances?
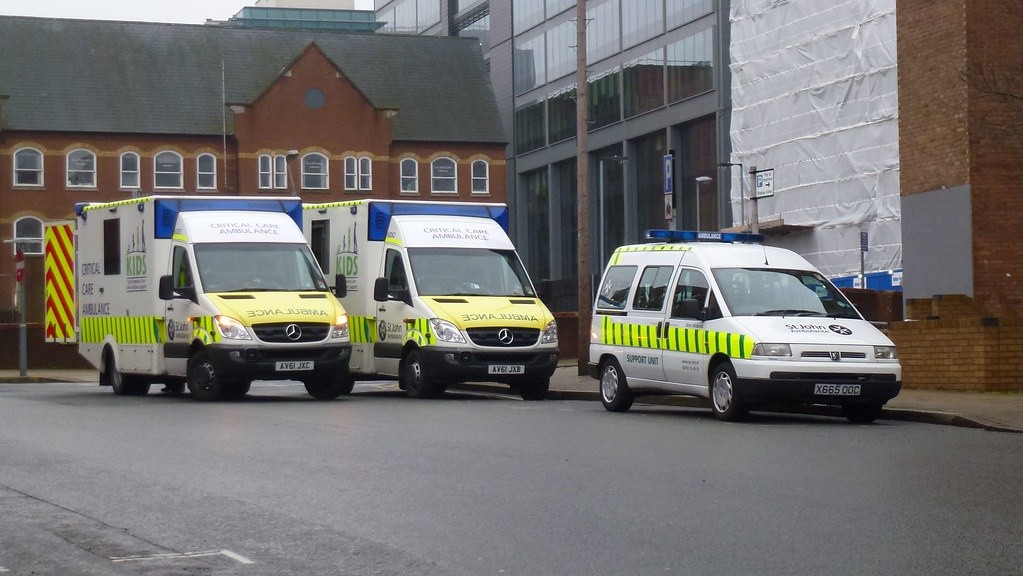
[41,192,351,401]
[301,198,559,400]
[586,229,903,423]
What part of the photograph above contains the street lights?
[600,156,628,278]
[718,162,744,223]
[696,176,713,231]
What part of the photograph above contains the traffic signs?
[754,168,774,199]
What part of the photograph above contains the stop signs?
[16,249,26,282]
